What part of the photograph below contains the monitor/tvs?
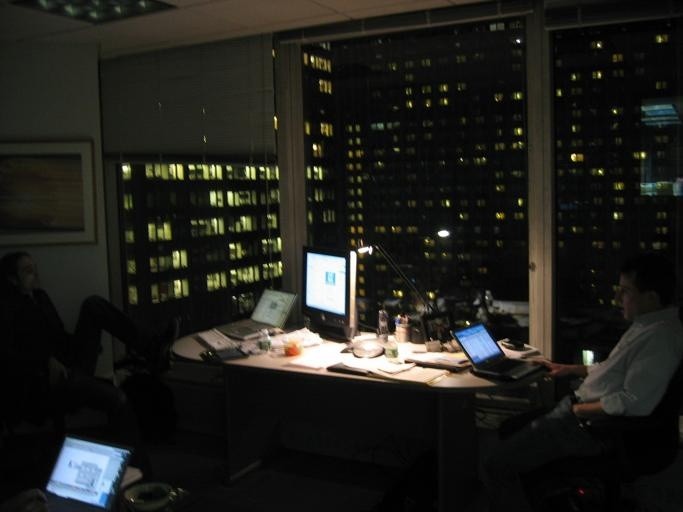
[301,244,362,343]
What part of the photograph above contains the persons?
[0,249,172,445]
[507,245,681,509]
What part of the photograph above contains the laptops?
[216,288,299,341]
[43,434,136,512]
[450,322,544,380]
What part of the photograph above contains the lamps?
[368,242,437,315]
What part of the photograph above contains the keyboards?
[197,328,237,352]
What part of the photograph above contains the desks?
[169,318,551,512]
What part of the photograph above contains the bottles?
[255,328,272,361]
[384,334,401,376]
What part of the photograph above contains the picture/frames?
[419,314,454,344]
[0,139,98,249]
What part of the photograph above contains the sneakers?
[158,318,179,368]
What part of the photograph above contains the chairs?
[542,358,682,511]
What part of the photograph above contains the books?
[267,325,474,389]
